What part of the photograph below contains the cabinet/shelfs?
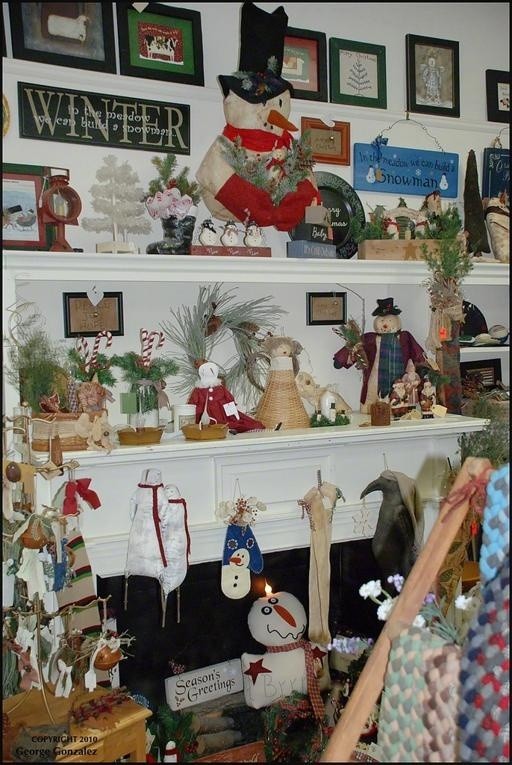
[0,247,508,587]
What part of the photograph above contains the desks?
[1,685,151,765]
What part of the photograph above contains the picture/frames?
[406,35,460,118]
[2,170,52,252]
[5,0,117,73]
[114,3,206,87]
[62,289,125,338]
[485,68,511,124]
[304,288,349,327]
[280,28,327,102]
[329,36,388,111]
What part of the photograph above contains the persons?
[0,1,511,711]
[3,1,509,710]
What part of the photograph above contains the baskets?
[22,408,111,451]
